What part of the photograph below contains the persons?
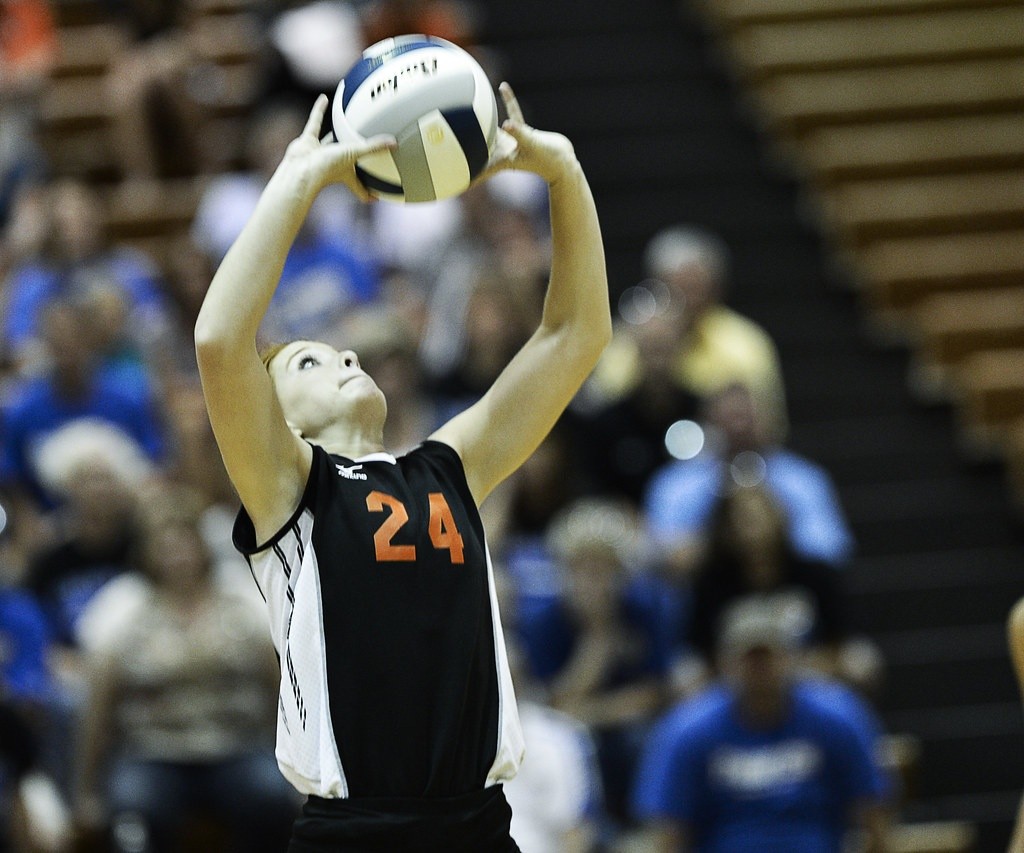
[192,82,614,853]
[0,0,302,853]
[197,0,890,853]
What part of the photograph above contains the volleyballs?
[331,31,501,204]
